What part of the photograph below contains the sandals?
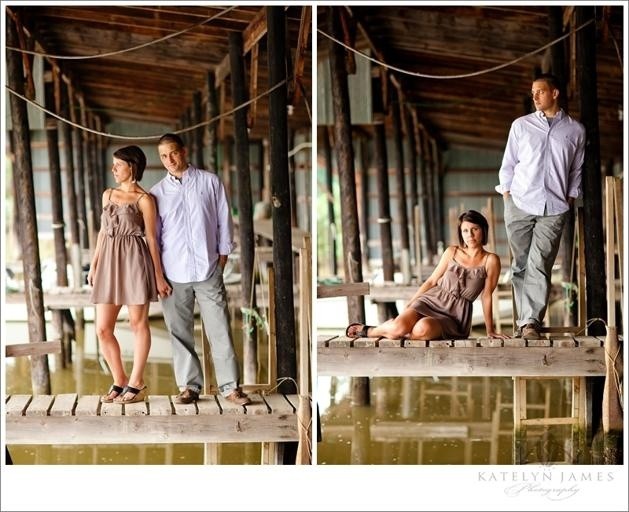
[346,323,376,338]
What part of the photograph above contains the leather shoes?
[225,389,250,405]
[174,390,199,403]
[523,324,540,340]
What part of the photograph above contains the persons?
[494,73,586,339]
[87,145,172,404]
[146,133,253,406]
[345,210,511,341]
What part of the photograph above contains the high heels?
[101,384,146,404]
